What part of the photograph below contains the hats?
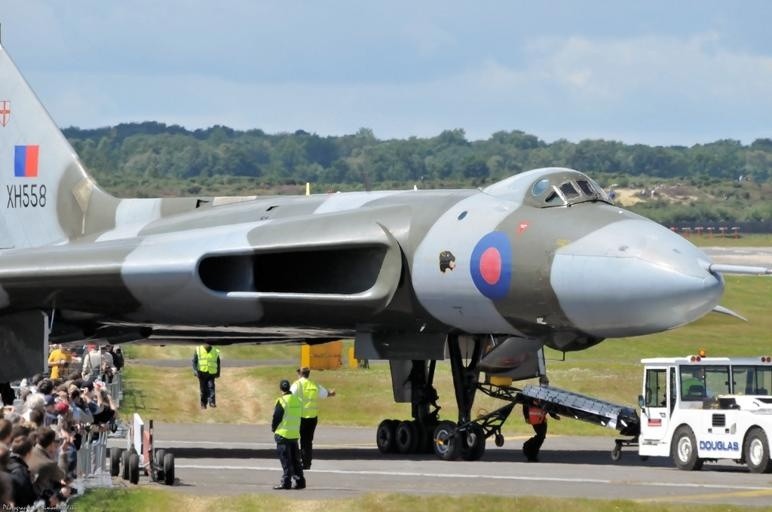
[55,402,69,413]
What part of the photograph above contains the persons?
[272,379,306,490]
[0,342,125,511]
[289,366,336,469]
[192,344,221,408]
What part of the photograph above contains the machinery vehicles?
[636,351,772,471]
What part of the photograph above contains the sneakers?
[273,477,306,490]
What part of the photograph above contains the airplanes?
[0,44,768,457]
